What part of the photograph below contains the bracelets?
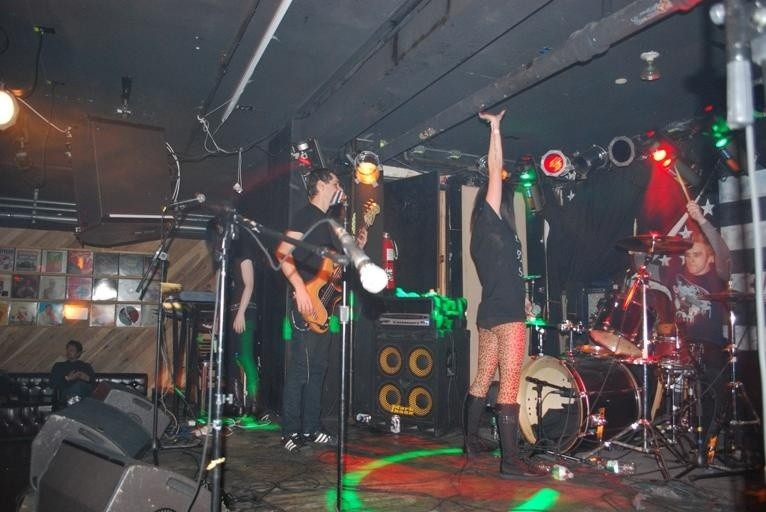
[490,126,500,135]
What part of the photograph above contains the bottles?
[535,461,577,483]
[590,458,637,475]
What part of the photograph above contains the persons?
[42,277,60,300]
[50,340,94,404]
[463,109,551,477]
[628,200,734,341]
[203,212,262,426]
[16,278,35,297]
[38,303,59,326]
[274,165,368,455]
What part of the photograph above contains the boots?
[462,392,500,454]
[495,401,551,481]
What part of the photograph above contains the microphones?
[332,226,388,295]
[166,193,207,211]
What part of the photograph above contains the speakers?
[92,376,171,443]
[37,437,224,512]
[30,396,152,488]
[72,116,172,248]
[375,329,453,437]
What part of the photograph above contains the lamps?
[535,134,653,181]
[639,50,662,82]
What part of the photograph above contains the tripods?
[586,289,689,480]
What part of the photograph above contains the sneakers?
[280,433,314,458]
[302,430,340,447]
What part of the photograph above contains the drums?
[516,355,642,453]
[654,335,689,362]
[587,295,656,356]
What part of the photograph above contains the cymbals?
[615,234,694,253]
[698,287,755,302]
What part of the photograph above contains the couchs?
[0,374,147,470]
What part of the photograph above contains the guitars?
[290,198,381,336]
